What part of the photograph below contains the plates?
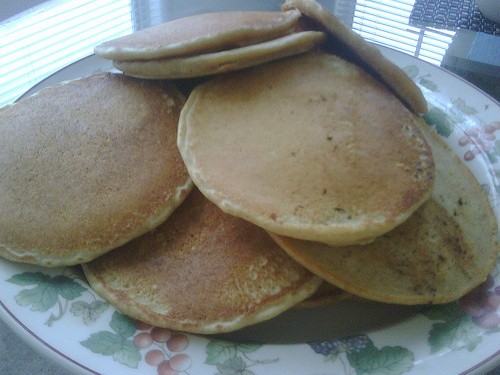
[1,39,500,374]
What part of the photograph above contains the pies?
[0,1,500,333]
